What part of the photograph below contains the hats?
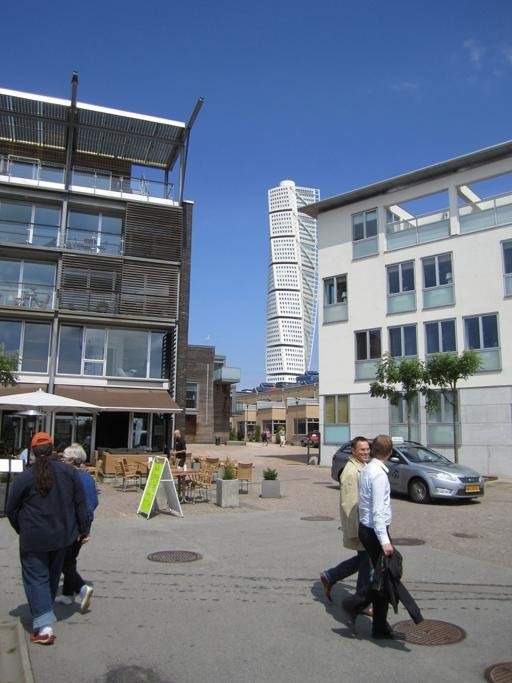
[31,431,54,447]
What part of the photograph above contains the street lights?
[245,403,250,442]
[295,396,300,446]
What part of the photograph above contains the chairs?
[115,366,127,376]
[73,234,109,253]
[129,172,147,195]
[78,449,254,504]
[13,286,51,307]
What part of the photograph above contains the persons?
[342,433,407,640]
[319,434,374,617]
[58,440,99,613]
[168,429,187,469]
[4,431,91,644]
[257,423,289,448]
[19,446,34,459]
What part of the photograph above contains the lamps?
[342,291,346,301]
[445,271,453,283]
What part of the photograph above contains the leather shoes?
[362,606,373,616]
[79,586,94,614]
[53,594,74,606]
[321,572,333,601]
[385,629,406,640]
[342,599,357,625]
[29,631,56,645]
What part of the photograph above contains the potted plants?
[96,300,109,313]
[260,467,281,499]
[216,463,241,509]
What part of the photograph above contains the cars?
[331,436,484,504]
[300,430,319,448]
